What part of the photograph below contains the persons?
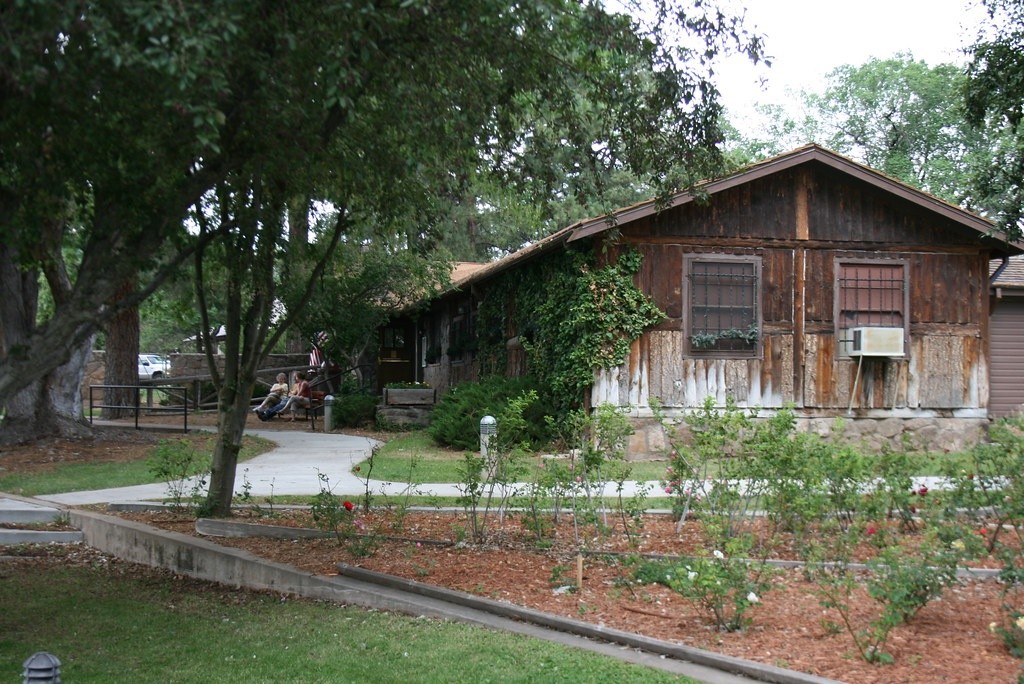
[253,372,312,423]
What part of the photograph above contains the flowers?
[384,380,435,389]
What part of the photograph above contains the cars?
[138,354,171,387]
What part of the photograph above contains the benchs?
[277,391,327,421]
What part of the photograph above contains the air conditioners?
[849,327,905,357]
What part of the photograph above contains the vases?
[381,388,437,405]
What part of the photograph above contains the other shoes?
[252,407,261,411]
[288,420,297,424]
[255,410,277,421]
[276,409,284,415]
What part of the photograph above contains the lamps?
[321,394,337,433]
[477,414,500,483]
